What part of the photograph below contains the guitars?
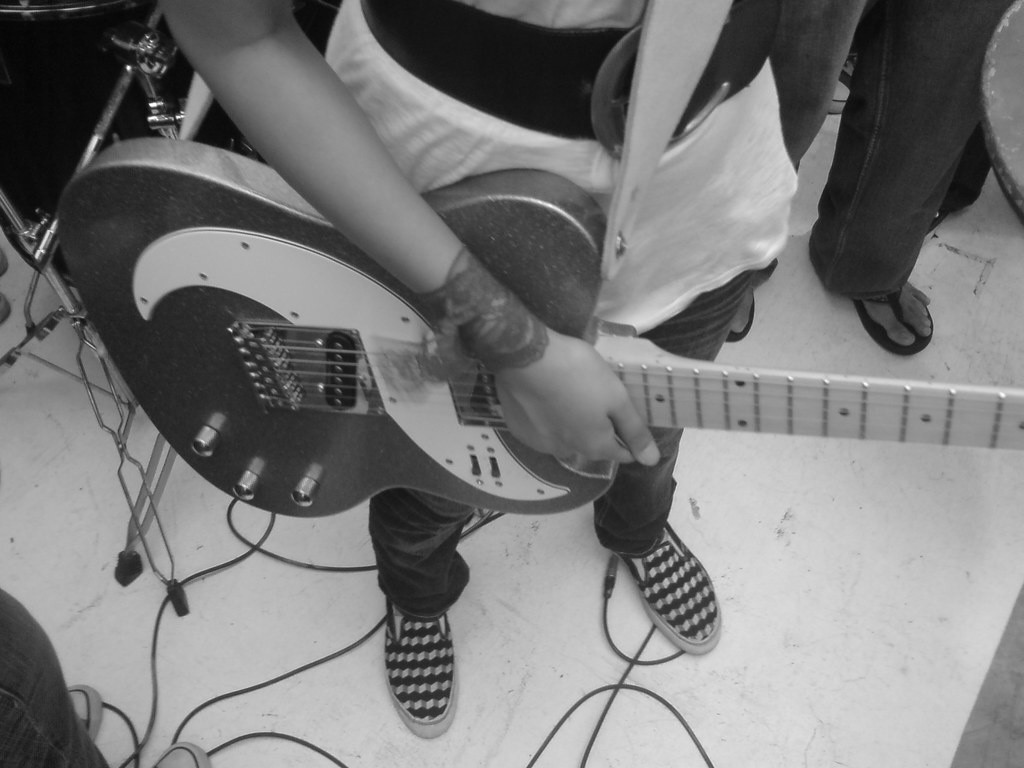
[54,137,1023,519]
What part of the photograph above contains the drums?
[980,0,1024,218]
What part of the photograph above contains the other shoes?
[619,520,722,655]
[384,599,457,739]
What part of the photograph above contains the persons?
[724,0,1017,356]
[0,589,210,768]
[161,0,798,741]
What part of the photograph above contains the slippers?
[726,290,754,342]
[853,283,935,356]
